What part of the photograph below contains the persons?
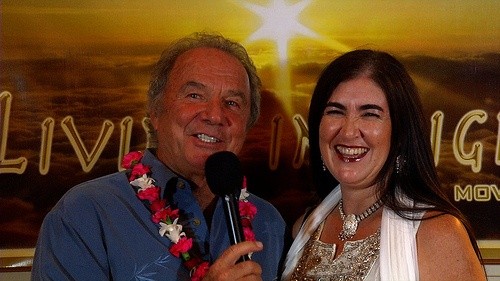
[276,50,488,281]
[32,28,285,281]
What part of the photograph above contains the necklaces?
[338,195,386,241]
[121,151,256,281]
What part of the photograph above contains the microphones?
[204,150,249,264]
